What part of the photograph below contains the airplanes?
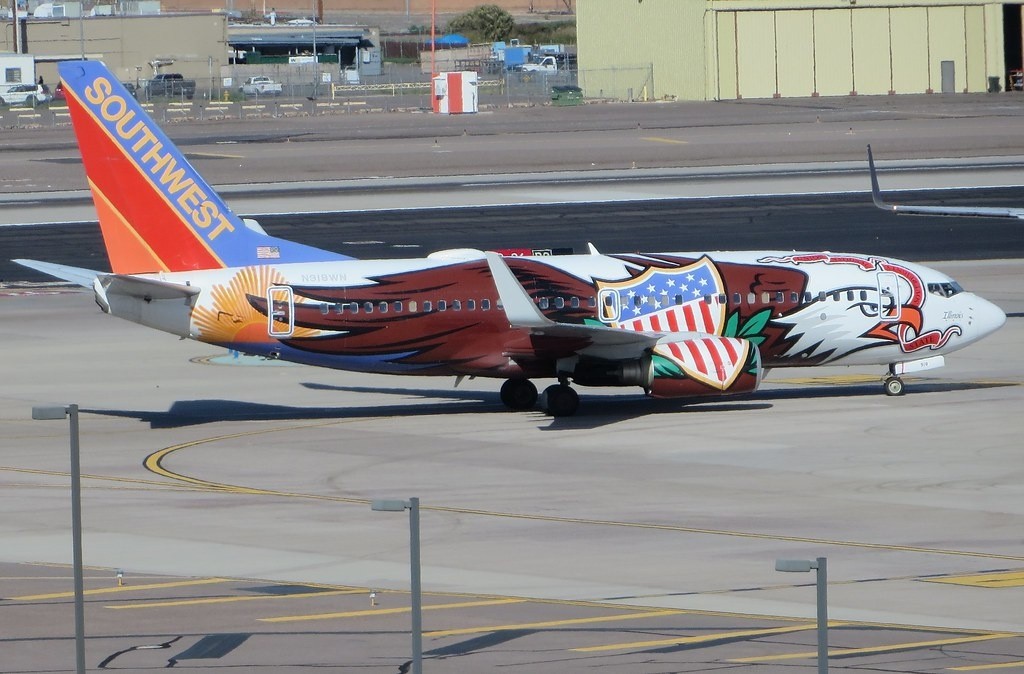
[7,59,1009,419]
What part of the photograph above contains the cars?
[123,83,137,99]
[53,81,65,100]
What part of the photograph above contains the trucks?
[521,56,578,77]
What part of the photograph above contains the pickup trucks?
[146,73,196,100]
[239,75,283,97]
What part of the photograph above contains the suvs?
[0,82,53,109]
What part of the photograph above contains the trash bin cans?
[345,66,360,84]
[552,86,584,107]
[988,76,1001,93]
